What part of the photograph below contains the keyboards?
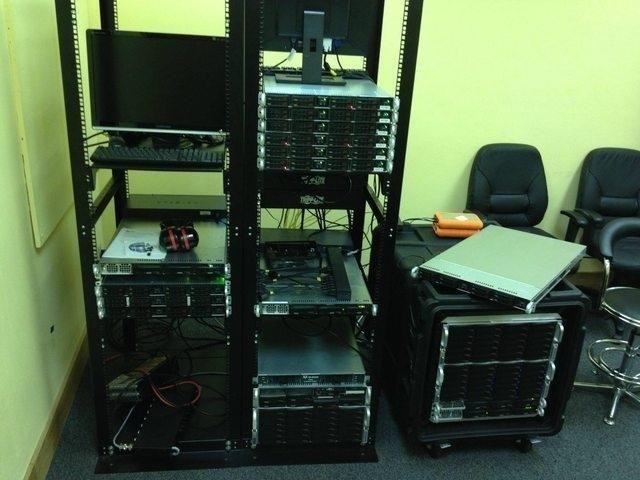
[88,146,226,169]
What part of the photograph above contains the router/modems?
[433,211,484,238]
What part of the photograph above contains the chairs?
[574,147,640,345]
[463,143,589,278]
[572,217,640,425]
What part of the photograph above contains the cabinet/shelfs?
[55,0,424,474]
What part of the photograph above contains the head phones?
[159,213,198,252]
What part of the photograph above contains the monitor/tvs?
[262,1,378,85]
[86,28,230,149]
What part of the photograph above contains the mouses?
[263,67,369,79]
